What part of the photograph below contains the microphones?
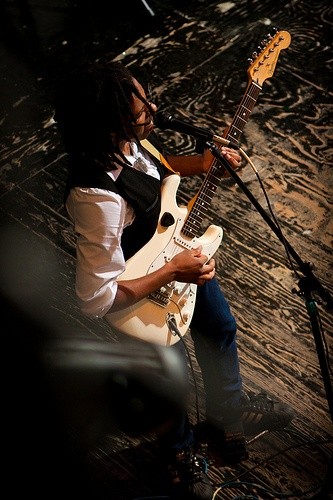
[155,110,239,150]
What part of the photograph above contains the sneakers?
[205,390,295,435]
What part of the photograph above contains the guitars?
[106,27,292,348]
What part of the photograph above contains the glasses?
[129,83,152,128]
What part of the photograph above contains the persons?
[60,62,290,441]
